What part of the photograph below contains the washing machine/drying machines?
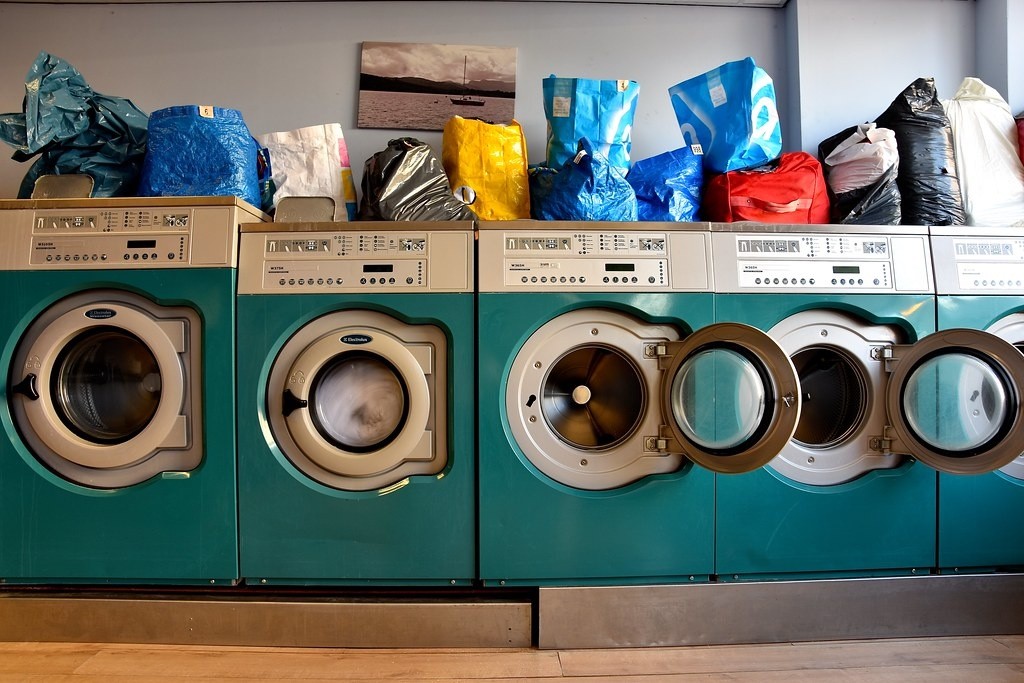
[923,225,1024,578]
[0,173,271,602]
[707,223,940,588]
[231,195,481,593]
[471,219,720,590]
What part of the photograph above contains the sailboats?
[450,55,486,106]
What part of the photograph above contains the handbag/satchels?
[137,105,270,209]
[357,138,477,220]
[628,146,701,220]
[444,115,529,220]
[823,122,899,193]
[541,74,640,176]
[667,55,783,171]
[706,151,829,224]
[530,139,641,221]
[251,123,359,220]
